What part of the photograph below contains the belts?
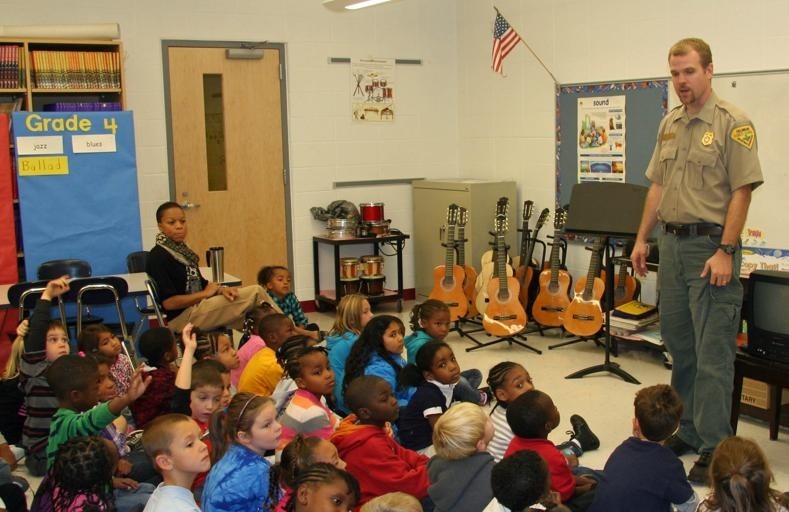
[666,223,723,237]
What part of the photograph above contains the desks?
[609,256,750,350]
[0,267,241,349]
[730,346,789,441]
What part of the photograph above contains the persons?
[148,202,328,346]
[1,267,600,510]
[586,384,700,511]
[629,37,764,488]
[697,435,788,512]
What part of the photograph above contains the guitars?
[428,197,641,338]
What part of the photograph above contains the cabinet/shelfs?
[0,37,127,257]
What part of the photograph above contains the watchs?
[720,243,738,258]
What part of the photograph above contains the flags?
[490,12,520,76]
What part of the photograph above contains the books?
[1,43,122,253]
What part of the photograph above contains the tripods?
[447,317,606,354]
[565,238,641,385]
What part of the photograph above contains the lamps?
[321,0,399,13]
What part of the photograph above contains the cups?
[208,246,223,282]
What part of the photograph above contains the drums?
[325,202,391,238]
[340,256,385,295]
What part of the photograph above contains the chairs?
[8,251,226,373]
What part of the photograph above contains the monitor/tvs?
[746,270,789,364]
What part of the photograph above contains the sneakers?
[687,450,716,486]
[570,413,600,451]
[663,434,692,456]
